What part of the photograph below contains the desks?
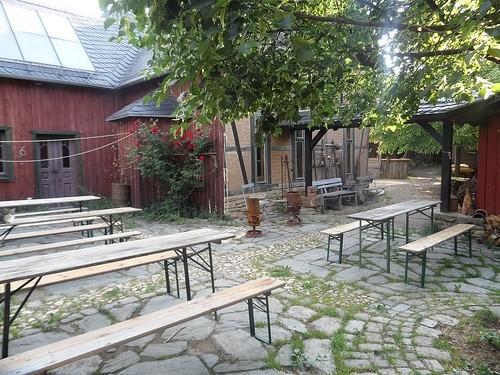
[0,195,100,238]
[0,207,142,249]
[345,200,443,274]
[0,228,237,360]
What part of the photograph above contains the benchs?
[398,224,476,287]
[322,216,395,264]
[0,206,195,360]
[310,177,359,213]
[1,277,286,375]
